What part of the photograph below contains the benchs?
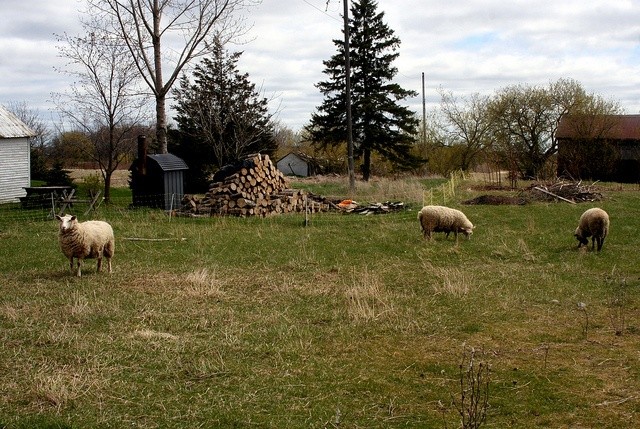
[16,196,63,206]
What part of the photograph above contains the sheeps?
[55,213,116,277]
[573,207,610,253]
[417,205,477,246]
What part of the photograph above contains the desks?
[21,183,71,206]
